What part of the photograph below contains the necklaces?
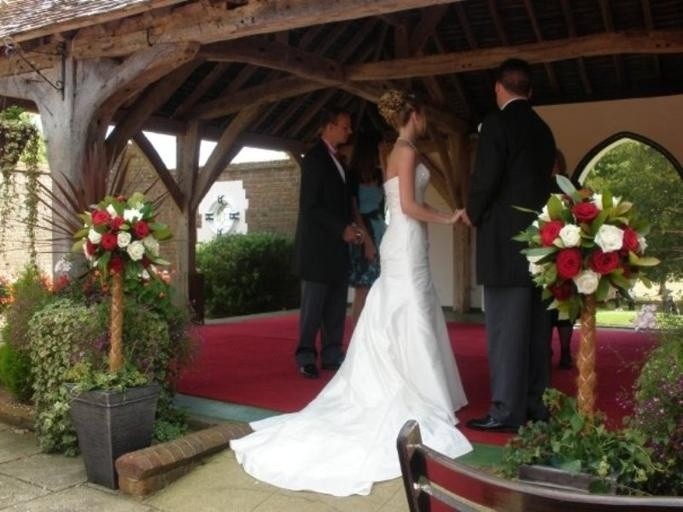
[397,135,417,152]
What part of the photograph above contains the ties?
[334,153,347,174]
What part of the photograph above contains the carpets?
[166,313,663,448]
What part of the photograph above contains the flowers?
[511,172,663,482]
[70,189,177,377]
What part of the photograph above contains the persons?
[549,147,589,373]
[294,105,377,379]
[455,58,559,433]
[228,86,476,498]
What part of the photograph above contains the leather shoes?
[321,358,342,369]
[465,412,522,431]
[299,362,319,378]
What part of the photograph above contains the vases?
[60,380,161,495]
